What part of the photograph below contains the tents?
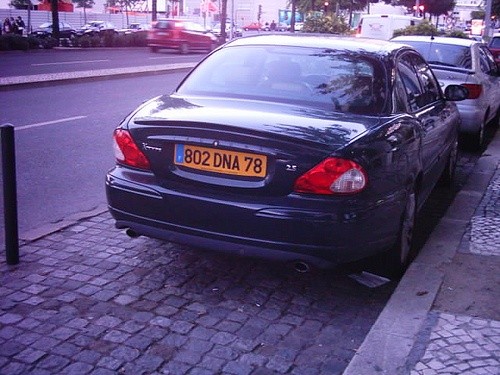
[24,0,123,14]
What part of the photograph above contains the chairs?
[326,73,365,98]
[258,61,313,94]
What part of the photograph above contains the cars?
[387,33,500,147]
[76,21,144,39]
[261,22,287,32]
[287,23,304,31]
[487,33,500,69]
[27,22,76,41]
[146,17,219,55]
[242,22,262,31]
[105,34,462,273]
[211,22,244,38]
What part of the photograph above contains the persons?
[263,20,276,31]
[0,16,25,35]
